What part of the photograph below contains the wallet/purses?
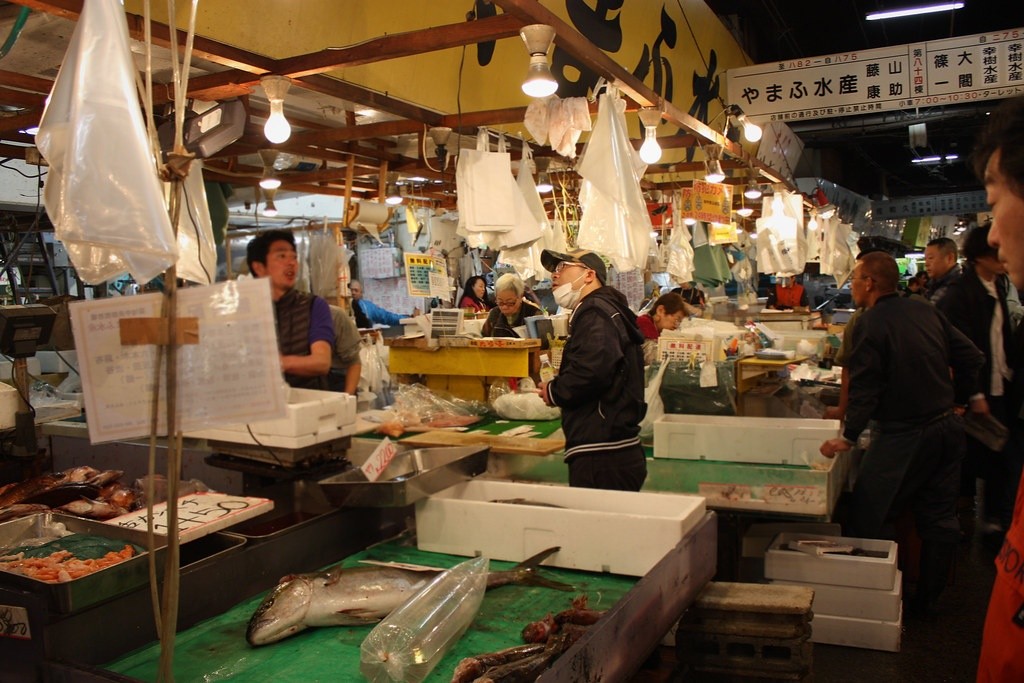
[964,412,1008,451]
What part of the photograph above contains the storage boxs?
[0,304,906,683]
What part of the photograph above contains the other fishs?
[1,463,142,520]
[448,598,601,683]
[246,544,575,653]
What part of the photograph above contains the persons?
[637,292,684,342]
[325,305,362,394]
[766,275,810,312]
[247,228,335,391]
[481,273,555,350]
[348,280,414,329]
[898,94,1024,683]
[821,252,986,622]
[671,282,705,317]
[536,249,648,492]
[458,276,492,313]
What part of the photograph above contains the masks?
[552,272,588,311]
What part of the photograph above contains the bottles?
[539,353,558,383]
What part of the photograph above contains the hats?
[540,249,607,281]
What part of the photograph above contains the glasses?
[551,261,585,272]
[848,274,878,283]
[495,299,517,307]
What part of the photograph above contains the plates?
[755,352,785,360]
